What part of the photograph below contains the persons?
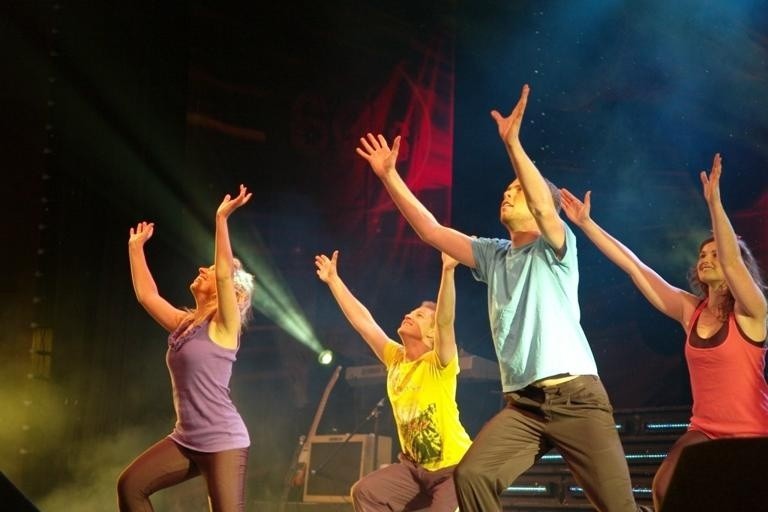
[313,232,475,511]
[115,181,259,511]
[352,81,642,510]
[556,151,768,510]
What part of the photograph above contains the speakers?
[303,435,393,504]
